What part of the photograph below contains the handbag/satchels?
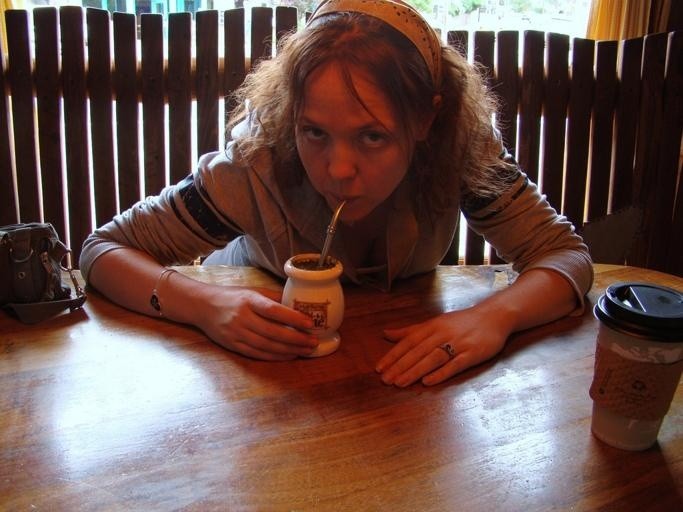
[0,222,88,325]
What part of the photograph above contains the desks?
[1,254,679,512]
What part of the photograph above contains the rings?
[438,343,456,359]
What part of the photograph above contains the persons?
[79,0,594,389]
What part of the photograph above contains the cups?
[588,281,683,451]
[281,253,345,358]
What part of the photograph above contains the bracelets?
[151,269,176,320]
